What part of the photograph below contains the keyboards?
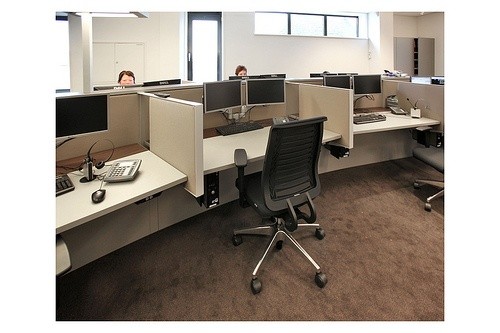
[56,174,74,196]
[353,114,386,124]
[216,122,264,136]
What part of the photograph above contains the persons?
[235,65,248,76]
[118,71,135,85]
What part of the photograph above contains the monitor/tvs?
[353,74,382,95]
[410,76,432,84]
[56,93,109,139]
[324,75,351,89]
[203,79,242,113]
[246,78,286,106]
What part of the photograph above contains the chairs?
[412,148,444,212]
[234,116,330,294]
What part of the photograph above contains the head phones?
[84,139,115,169]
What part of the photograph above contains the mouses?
[92,190,105,203]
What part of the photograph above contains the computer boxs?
[200,171,219,208]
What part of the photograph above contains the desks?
[56,71,443,285]
